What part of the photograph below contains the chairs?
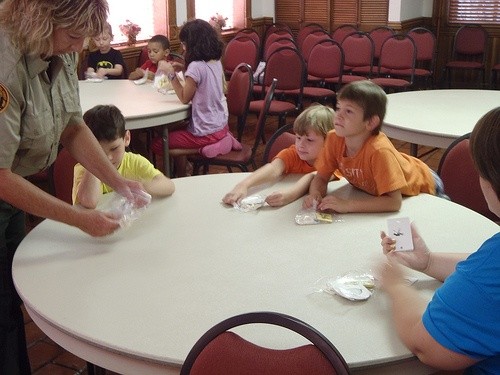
[137,24,489,175]
[177,312,351,374]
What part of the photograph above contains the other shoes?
[202,136,233,158]
[227,132,242,150]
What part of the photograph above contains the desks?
[371,80,500,150]
[11,173,500,374]
[77,76,192,179]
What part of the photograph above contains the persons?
[73,105,174,210]
[77,20,243,178]
[0,0,153,375]
[380,107,500,375]
[223,80,451,212]
